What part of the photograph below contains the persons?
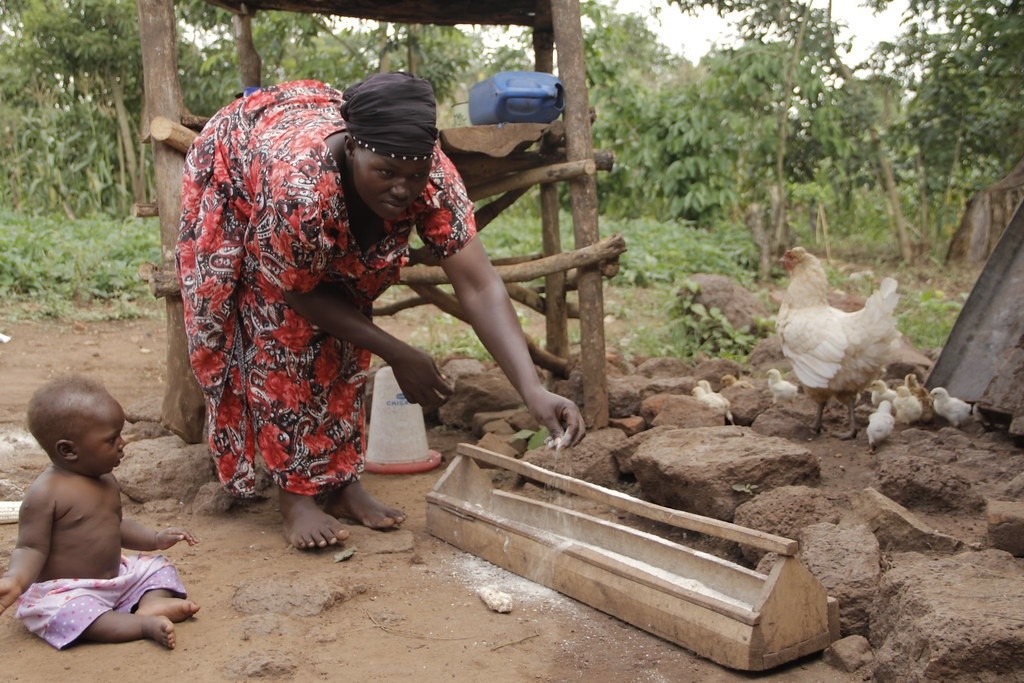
[1,374,201,651]
[175,70,586,549]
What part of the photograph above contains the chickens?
[691,379,735,425]
[720,374,754,391]
[824,373,1003,456]
[774,246,902,441]
[764,368,799,412]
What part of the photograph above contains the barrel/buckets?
[467,69,566,126]
